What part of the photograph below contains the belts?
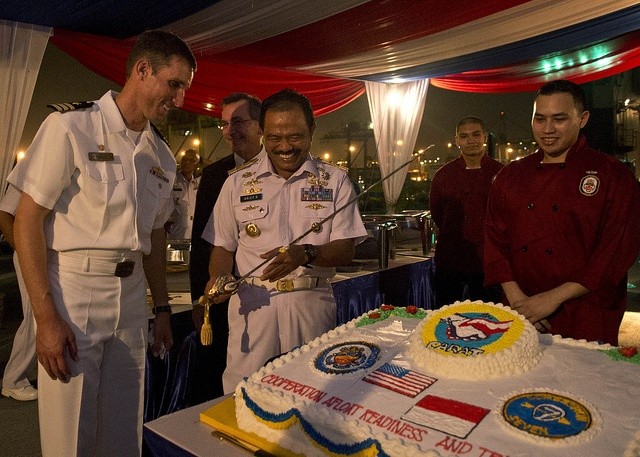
[54,254,144,277]
[244,277,327,291]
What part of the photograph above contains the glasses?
[218,117,254,129]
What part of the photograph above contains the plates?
[337,266,362,272]
[363,265,380,270]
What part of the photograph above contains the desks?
[144,391,265,456]
[329,251,432,325]
[384,238,436,275]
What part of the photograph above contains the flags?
[363,362,440,399]
[400,394,491,440]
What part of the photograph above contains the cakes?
[235,299,639,455]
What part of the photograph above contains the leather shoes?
[2,386,37,401]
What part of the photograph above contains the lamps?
[17,150,25,159]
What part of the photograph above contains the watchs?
[302,243,318,266]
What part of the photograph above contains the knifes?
[210,430,273,457]
[193,142,435,348]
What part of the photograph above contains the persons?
[151,305,174,314]
[0,182,39,402]
[190,93,263,404]
[483,79,637,350]
[205,87,357,395]
[5,29,198,453]
[164,149,202,239]
[429,117,505,308]
